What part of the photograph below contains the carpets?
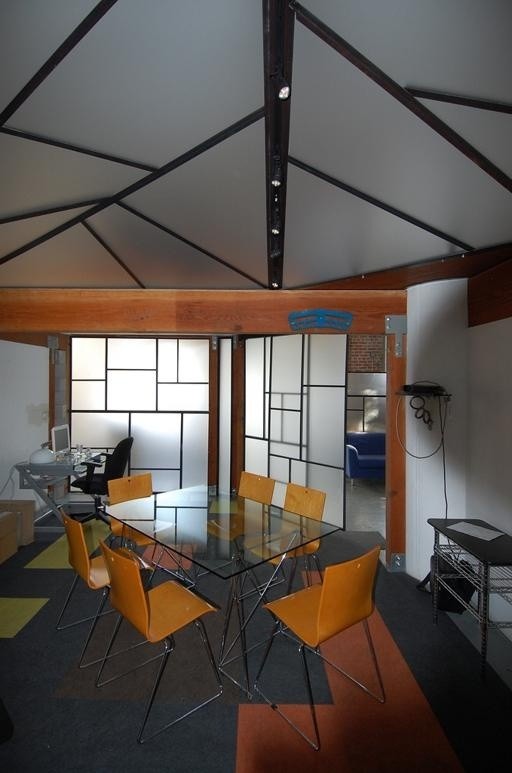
[0,512,512,773]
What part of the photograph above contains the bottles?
[85,447,92,460]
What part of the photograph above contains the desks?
[427,519,512,674]
[15,450,101,534]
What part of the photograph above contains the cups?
[76,443,83,454]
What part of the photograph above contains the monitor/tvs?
[51,424,70,456]
